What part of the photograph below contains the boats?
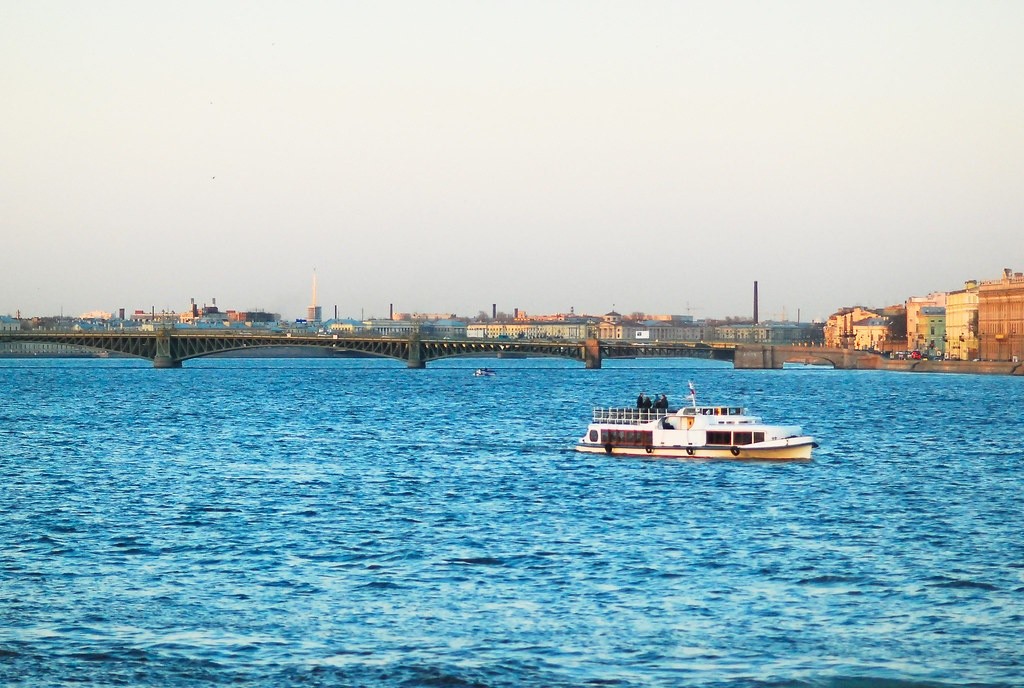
[572,380,818,463]
[472,369,496,376]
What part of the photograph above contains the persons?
[637,393,668,413]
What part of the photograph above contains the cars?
[869,346,992,362]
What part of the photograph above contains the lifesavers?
[604,442,612,453]
[686,446,694,455]
[645,444,652,454]
[730,446,740,456]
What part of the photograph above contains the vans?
[499,335,511,342]
